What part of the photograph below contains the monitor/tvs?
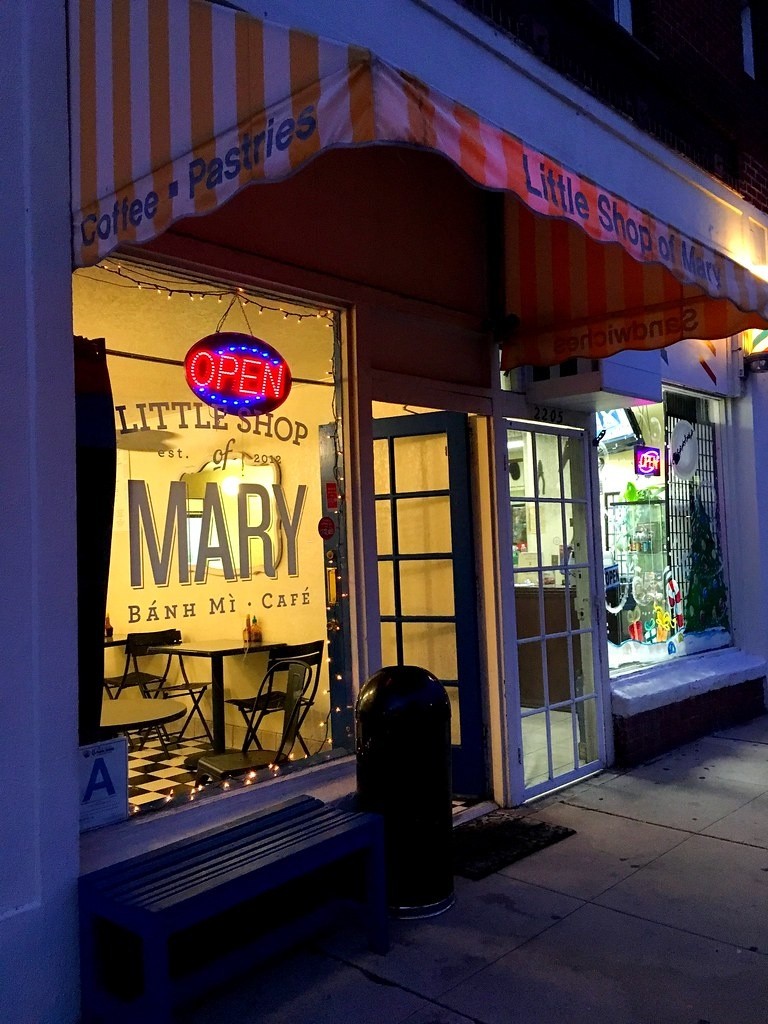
[596,406,646,455]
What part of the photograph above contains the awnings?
[71,1,767,367]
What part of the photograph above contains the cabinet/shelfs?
[513,582,621,713]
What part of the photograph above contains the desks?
[99,698,187,741]
[147,640,287,774]
[104,633,129,649]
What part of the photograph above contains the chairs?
[103,627,177,749]
[195,661,313,791]
[130,630,215,758]
[224,639,325,757]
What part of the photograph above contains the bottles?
[250,616,262,642]
[105,613,113,637]
[243,614,251,642]
[521,544,526,552]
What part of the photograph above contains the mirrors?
[177,451,282,577]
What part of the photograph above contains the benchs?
[77,795,389,1024]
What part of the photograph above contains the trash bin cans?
[354,665,461,920]
